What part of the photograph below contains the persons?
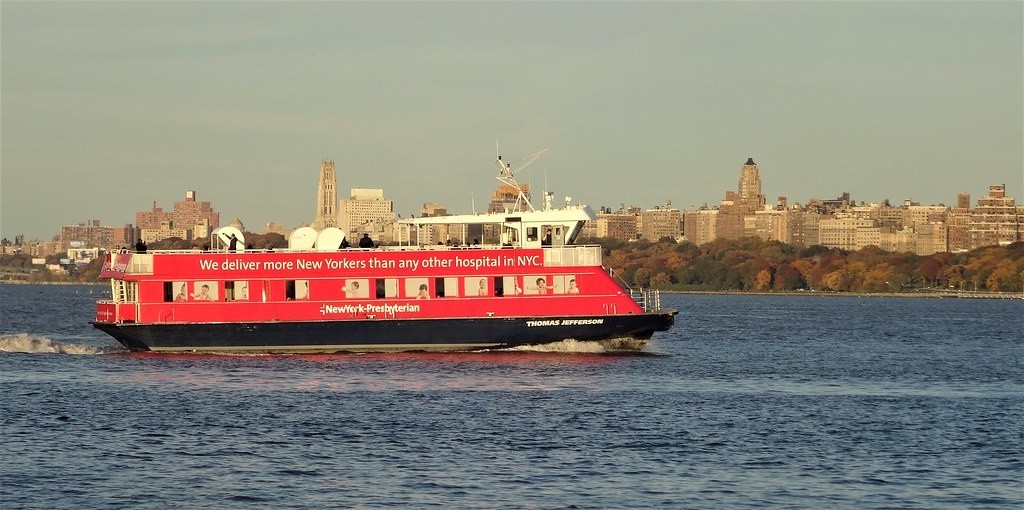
[177,284,187,302]
[301,282,308,299]
[528,277,557,294]
[514,276,522,296]
[190,285,214,301]
[237,287,247,300]
[341,281,359,297]
[478,278,486,294]
[339,228,379,253]
[567,279,580,293]
[117,234,275,254]
[417,284,430,300]
[418,229,552,250]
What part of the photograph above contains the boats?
[86,139,682,360]
